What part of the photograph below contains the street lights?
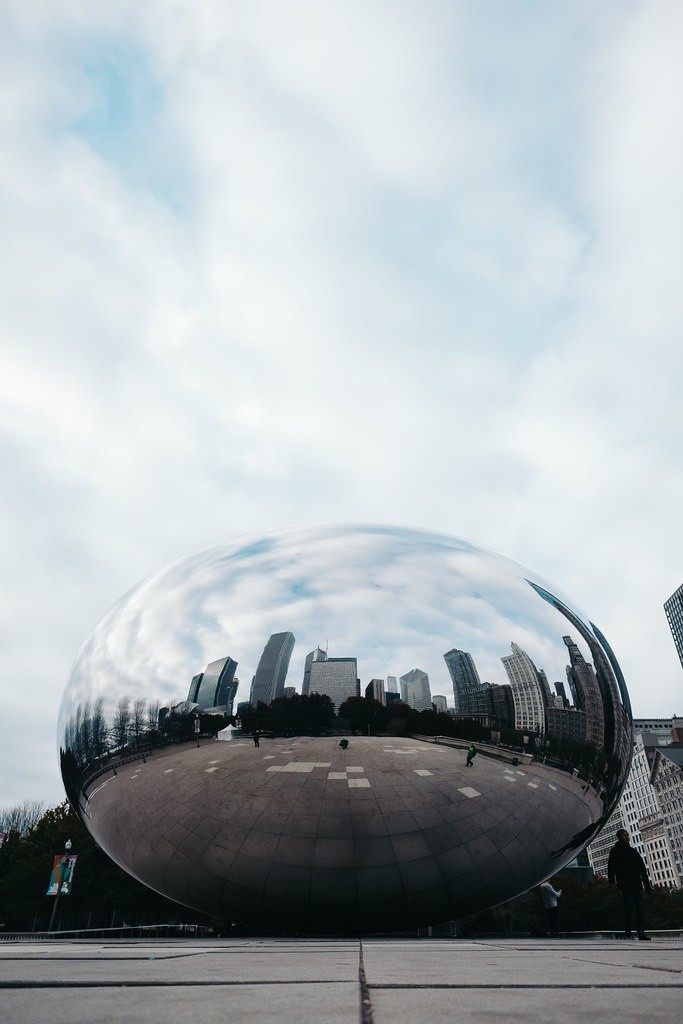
[49,839,73,930]
[195,712,201,747]
[236,715,242,738]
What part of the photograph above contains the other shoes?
[639,936,651,940]
[625,930,634,940]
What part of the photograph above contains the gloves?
[645,887,655,896]
[617,879,624,888]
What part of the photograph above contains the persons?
[464,744,477,768]
[608,830,651,941]
[540,879,563,937]
[253,733,260,746]
